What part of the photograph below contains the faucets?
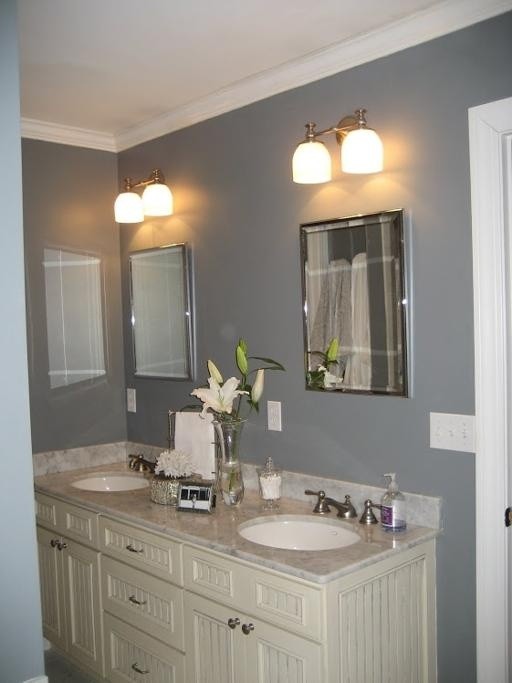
[128,453,159,475]
[321,494,358,519]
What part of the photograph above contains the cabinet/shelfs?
[34,488,105,682]
[98,511,186,682]
[181,537,438,682]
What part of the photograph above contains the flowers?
[179,337,286,419]
[307,337,346,389]
[155,449,199,478]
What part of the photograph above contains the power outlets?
[127,387,137,413]
[266,400,281,431]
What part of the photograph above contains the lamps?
[292,107,385,184]
[113,167,173,223]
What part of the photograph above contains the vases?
[150,472,202,505]
[211,416,248,508]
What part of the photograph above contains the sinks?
[230,512,364,553]
[67,472,151,493]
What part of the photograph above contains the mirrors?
[126,241,197,382]
[40,242,109,398]
[299,208,413,400]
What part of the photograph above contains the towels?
[174,410,216,479]
